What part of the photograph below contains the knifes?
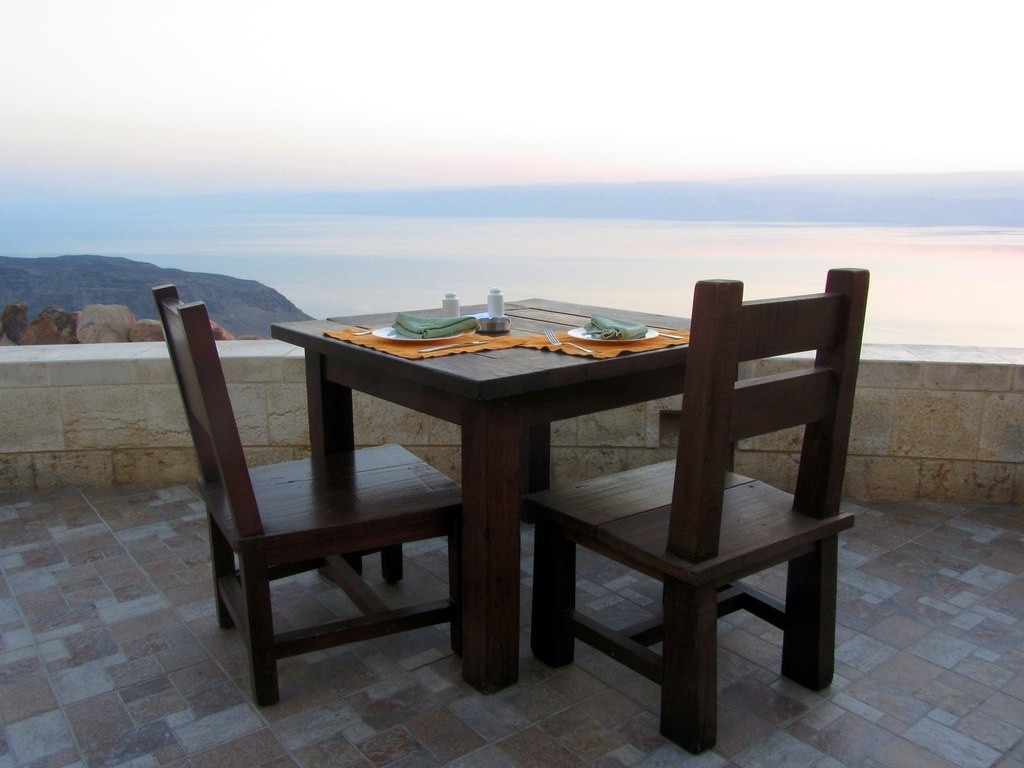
[416,340,492,353]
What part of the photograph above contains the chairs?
[148,284,462,707]
[510,266,873,756]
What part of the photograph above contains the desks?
[269,295,692,693]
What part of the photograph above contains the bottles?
[487,288,505,319]
[443,292,460,320]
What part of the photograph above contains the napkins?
[582,314,648,340]
[392,313,480,339]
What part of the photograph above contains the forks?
[544,328,594,352]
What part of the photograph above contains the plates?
[567,327,659,343]
[371,327,475,342]
